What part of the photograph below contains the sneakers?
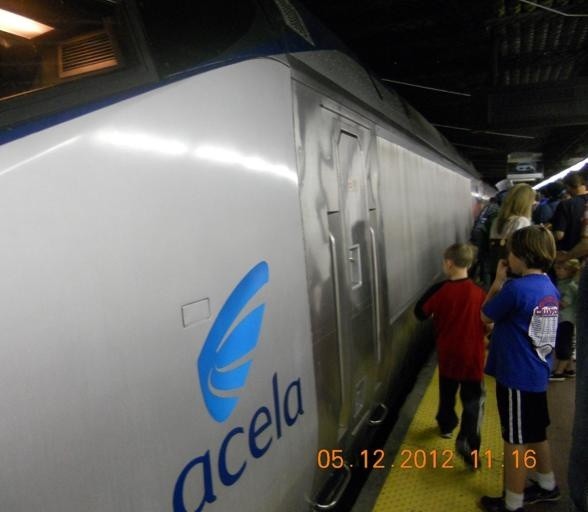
[456,440,482,469]
[547,373,565,382]
[524,482,561,506]
[440,427,452,439]
[479,496,524,512]
[564,369,577,376]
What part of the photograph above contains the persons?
[468,171,588,383]
[413,243,495,468]
[473,222,563,512]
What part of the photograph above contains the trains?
[0,1,500,512]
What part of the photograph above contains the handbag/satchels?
[486,220,523,277]
